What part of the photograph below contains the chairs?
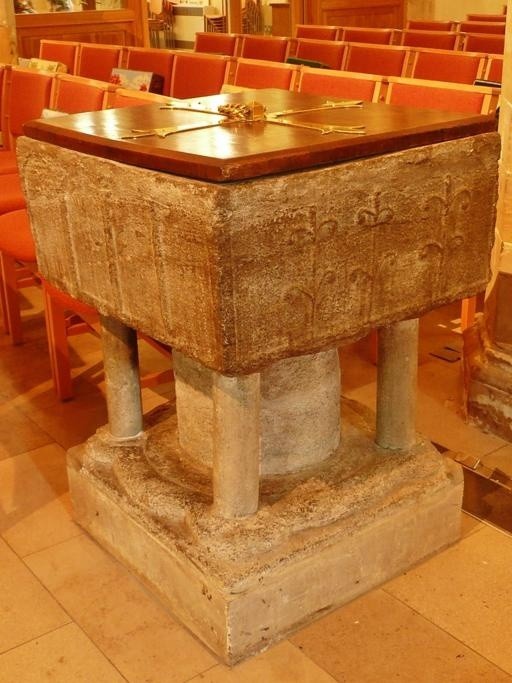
[1,0,509,403]
